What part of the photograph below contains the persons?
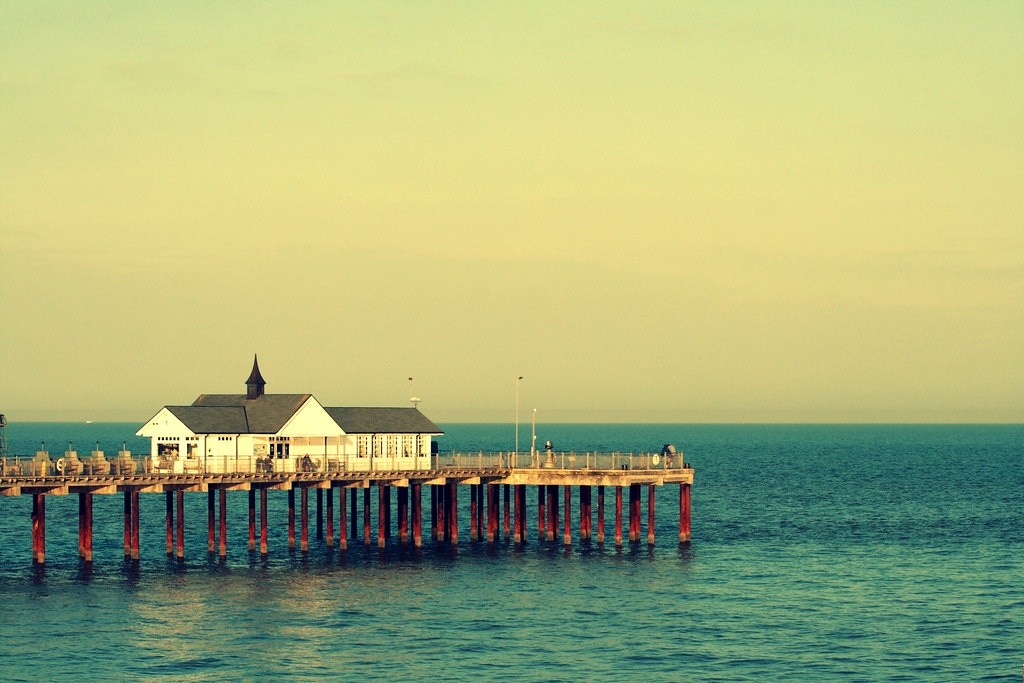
[256,454,317,475]
[661,444,676,469]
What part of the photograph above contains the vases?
[303,465,310,472]
[154,460,170,469]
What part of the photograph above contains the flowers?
[156,454,169,461]
[296,454,317,468]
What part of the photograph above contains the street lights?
[515,375,523,468]
[408,376,414,407]
[530,408,537,468]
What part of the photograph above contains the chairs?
[117,451,138,475]
[61,450,83,474]
[31,451,53,475]
[90,451,111,474]
